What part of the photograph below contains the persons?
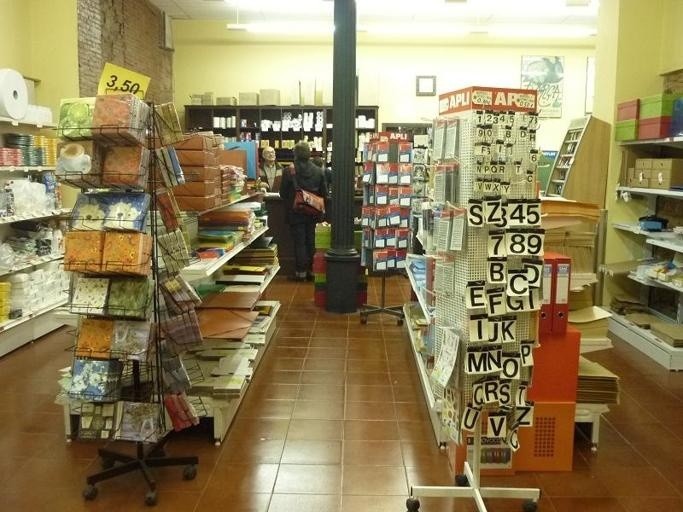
[311,157,331,186]
[257,145,285,195]
[278,140,331,283]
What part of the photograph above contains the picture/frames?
[415,75,437,96]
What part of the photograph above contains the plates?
[54,155,91,180]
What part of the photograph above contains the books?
[55,93,280,445]
[538,191,682,407]
[0,132,56,168]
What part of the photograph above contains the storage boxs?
[616,94,673,141]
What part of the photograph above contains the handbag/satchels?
[292,189,325,216]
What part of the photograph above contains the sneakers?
[288,273,315,282]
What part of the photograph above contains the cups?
[60,144,90,172]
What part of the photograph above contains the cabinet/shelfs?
[178,105,381,284]
[0,115,77,358]
[53,109,284,450]
[542,116,611,211]
[600,138,683,376]
